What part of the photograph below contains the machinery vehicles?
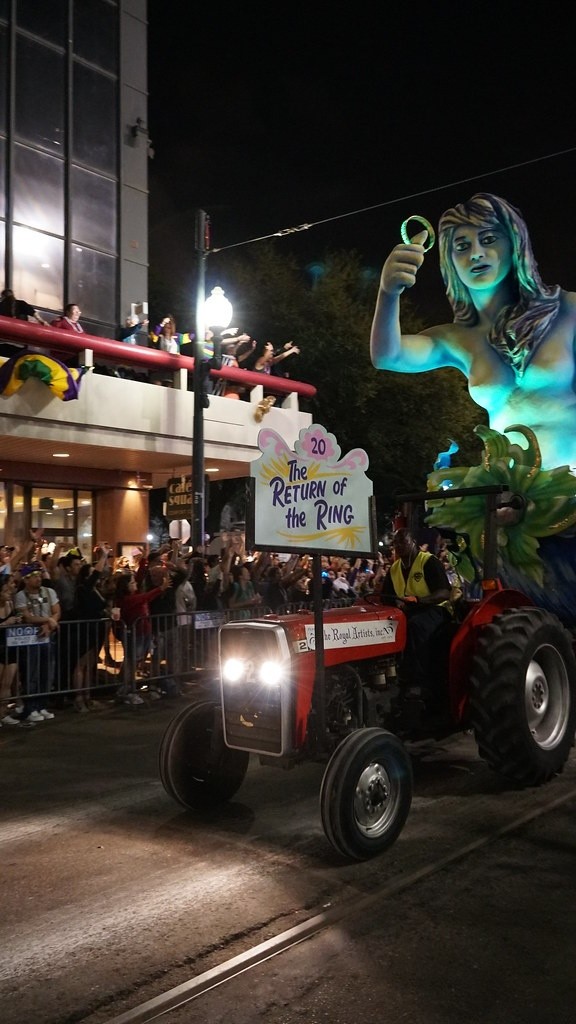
[158,486,576,861]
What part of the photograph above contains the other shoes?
[40,710,55,720]
[124,693,145,705]
[74,701,89,713]
[26,711,44,721]
[2,716,19,724]
[88,701,107,711]
[148,691,160,700]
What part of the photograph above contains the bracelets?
[401,215,436,253]
[31,538,36,543]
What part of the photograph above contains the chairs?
[424,587,470,702]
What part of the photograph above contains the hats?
[338,559,350,568]
[21,565,41,580]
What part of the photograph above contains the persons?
[0,529,462,725]
[0,288,299,401]
[369,192,575,471]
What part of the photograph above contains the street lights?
[192,286,236,557]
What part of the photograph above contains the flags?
[0,350,90,402]
